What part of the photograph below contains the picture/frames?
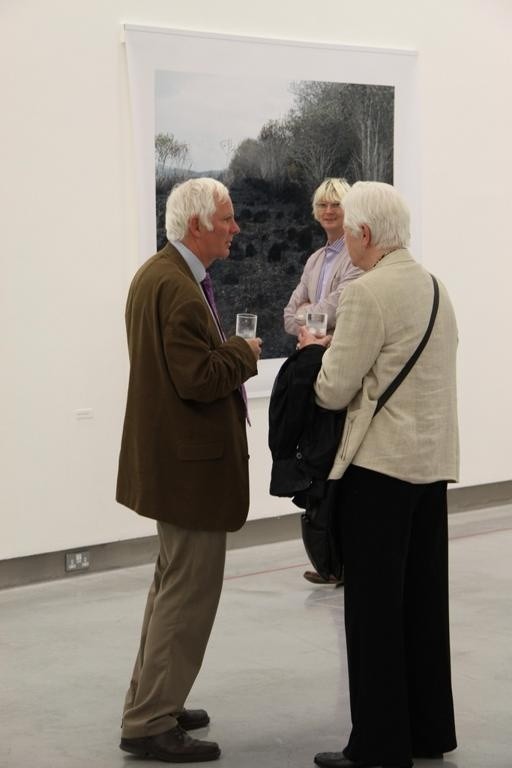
[124,24,416,399]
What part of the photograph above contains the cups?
[308,313,327,339]
[235,312,258,341]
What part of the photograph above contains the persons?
[281,176,352,589]
[312,180,463,768]
[114,169,265,764]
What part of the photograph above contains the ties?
[202,272,251,427]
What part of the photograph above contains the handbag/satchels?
[301,489,341,581]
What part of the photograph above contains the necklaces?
[369,248,408,270]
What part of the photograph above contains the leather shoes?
[315,751,413,768]
[176,710,209,730]
[121,727,220,762]
